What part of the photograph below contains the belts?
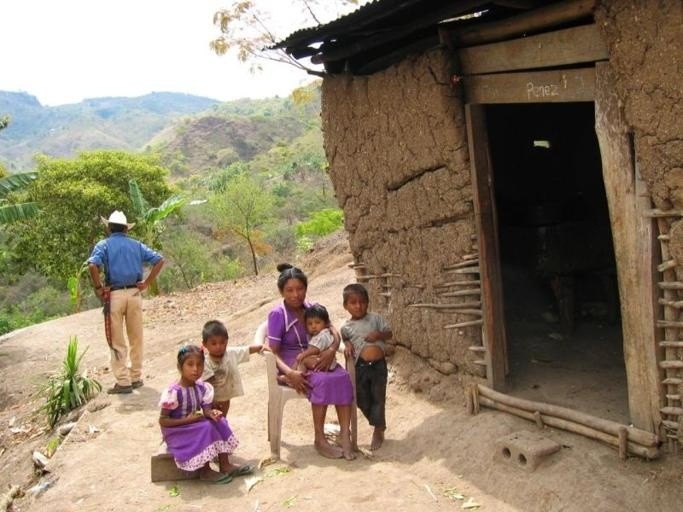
[110,282,137,292]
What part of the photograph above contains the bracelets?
[96,285,101,289]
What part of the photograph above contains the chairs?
[254,320,358,458]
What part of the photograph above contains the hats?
[101,209,136,231]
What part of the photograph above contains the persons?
[159,345,255,485]
[200,320,271,418]
[277,304,337,387]
[267,262,354,461]
[340,284,393,450]
[87,210,164,394]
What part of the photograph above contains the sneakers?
[107,380,144,394]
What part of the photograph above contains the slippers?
[199,463,252,483]
